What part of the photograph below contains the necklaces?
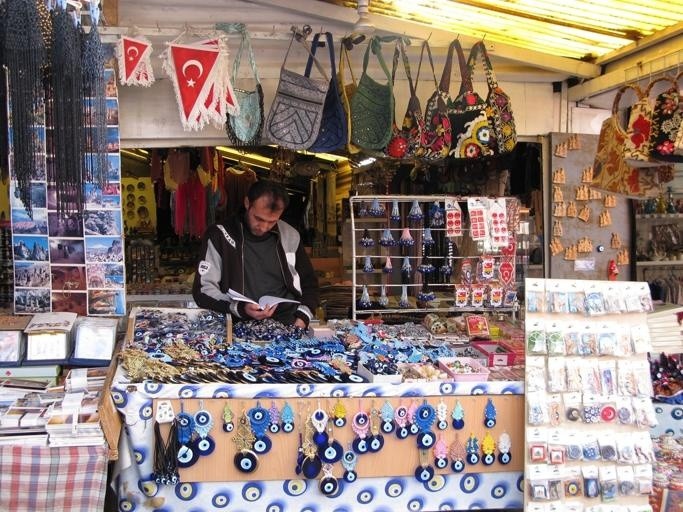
[153,418,192,485]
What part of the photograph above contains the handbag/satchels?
[226,30,518,159]
[591,73,683,199]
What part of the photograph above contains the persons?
[193,181,320,331]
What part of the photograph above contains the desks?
[0,306,683,512]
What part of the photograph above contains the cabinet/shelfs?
[636,212,683,268]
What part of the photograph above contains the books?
[0,313,120,449]
[226,288,300,311]
